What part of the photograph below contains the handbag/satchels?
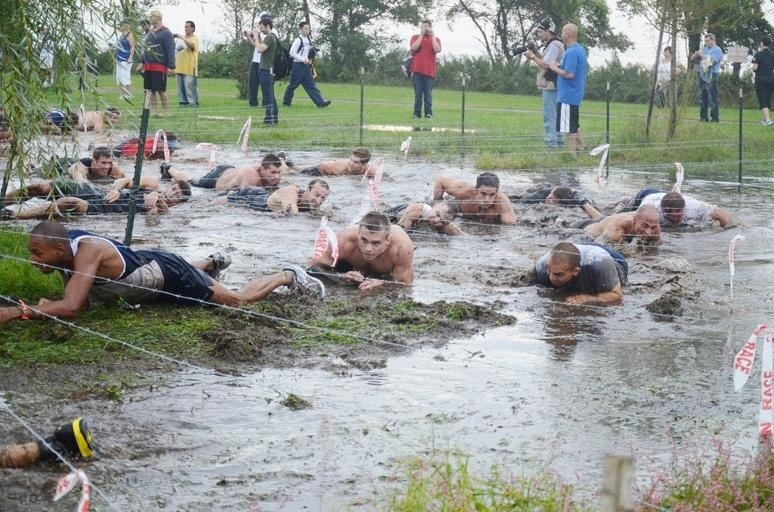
[401,56,414,81]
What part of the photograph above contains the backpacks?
[286,35,312,75]
[270,32,290,81]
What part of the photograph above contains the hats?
[159,131,180,149]
[117,19,131,26]
[536,19,556,35]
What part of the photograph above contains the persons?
[277,146,380,176]
[308,210,414,289]
[173,21,200,106]
[113,21,135,100]
[569,204,663,246]
[690,33,722,123]
[216,178,330,214]
[548,23,587,157]
[143,10,176,111]
[252,18,280,124]
[1,418,94,469]
[244,14,278,106]
[1,222,325,325]
[383,199,467,235]
[136,19,150,75]
[39,28,54,88]
[283,22,332,108]
[1,112,14,140]
[434,171,518,226]
[751,37,774,127]
[25,146,129,183]
[514,186,601,218]
[1,176,191,220]
[523,21,565,149]
[88,130,178,161]
[410,21,441,120]
[607,188,731,228]
[160,153,283,192]
[35,108,120,135]
[654,47,686,121]
[532,241,628,305]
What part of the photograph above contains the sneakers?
[208,249,231,279]
[607,198,630,209]
[761,120,773,127]
[276,151,286,160]
[570,190,586,205]
[54,417,98,462]
[317,100,331,107]
[285,160,293,166]
[283,263,325,302]
[160,160,172,180]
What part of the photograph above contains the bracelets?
[18,299,29,319]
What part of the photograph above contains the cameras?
[250,33,260,38]
[691,55,701,62]
[174,34,177,37]
[512,41,537,56]
